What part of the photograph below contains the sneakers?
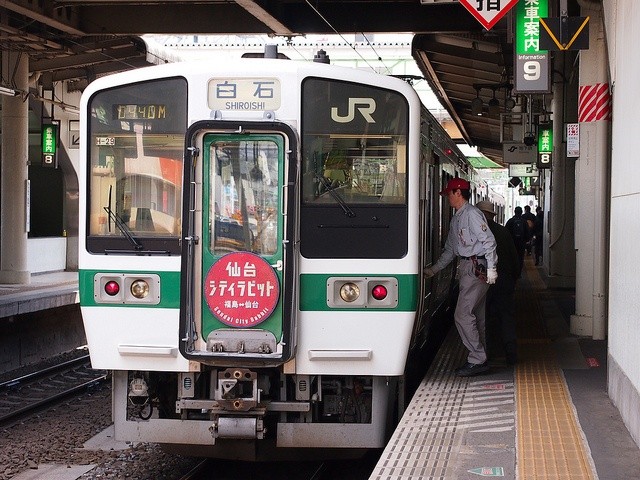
[457,364,489,376]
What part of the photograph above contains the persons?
[505,206,524,280]
[425,177,499,377]
[470,200,509,339]
[534,207,544,267]
[521,205,535,257]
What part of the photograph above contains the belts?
[460,256,486,260]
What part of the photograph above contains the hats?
[475,201,498,215]
[438,178,469,195]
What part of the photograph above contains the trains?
[75,46,509,464]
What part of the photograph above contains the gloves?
[423,268,433,279]
[486,269,498,284]
